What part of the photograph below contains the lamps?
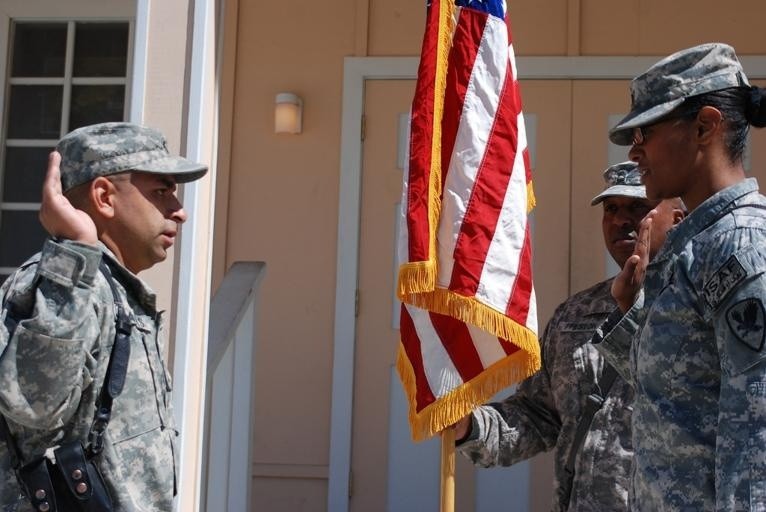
[274,92,303,136]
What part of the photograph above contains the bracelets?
[453,416,474,447]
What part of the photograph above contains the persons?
[1,122,209,511]
[451,158,688,511]
[589,41,766,512]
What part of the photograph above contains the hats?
[55,122,208,195]
[609,41,753,147]
[590,160,646,207]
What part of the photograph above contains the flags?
[389,0,544,446]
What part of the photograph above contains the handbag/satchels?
[19,442,113,512]
